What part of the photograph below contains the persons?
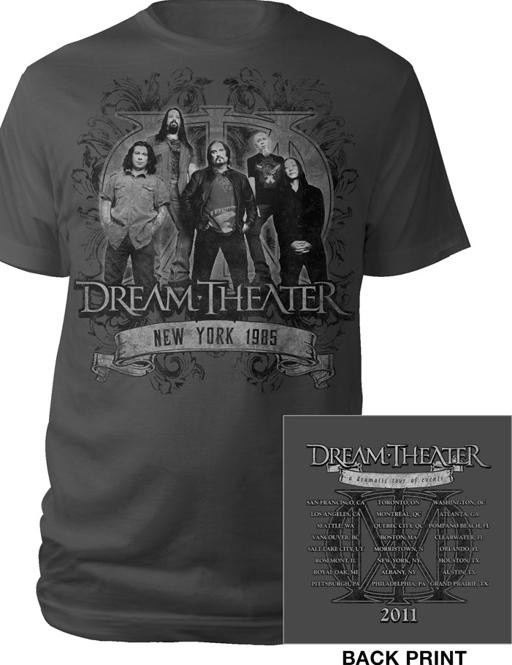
[245,129,286,289]
[272,154,328,284]
[146,107,193,285]
[178,140,259,304]
[99,141,172,297]
